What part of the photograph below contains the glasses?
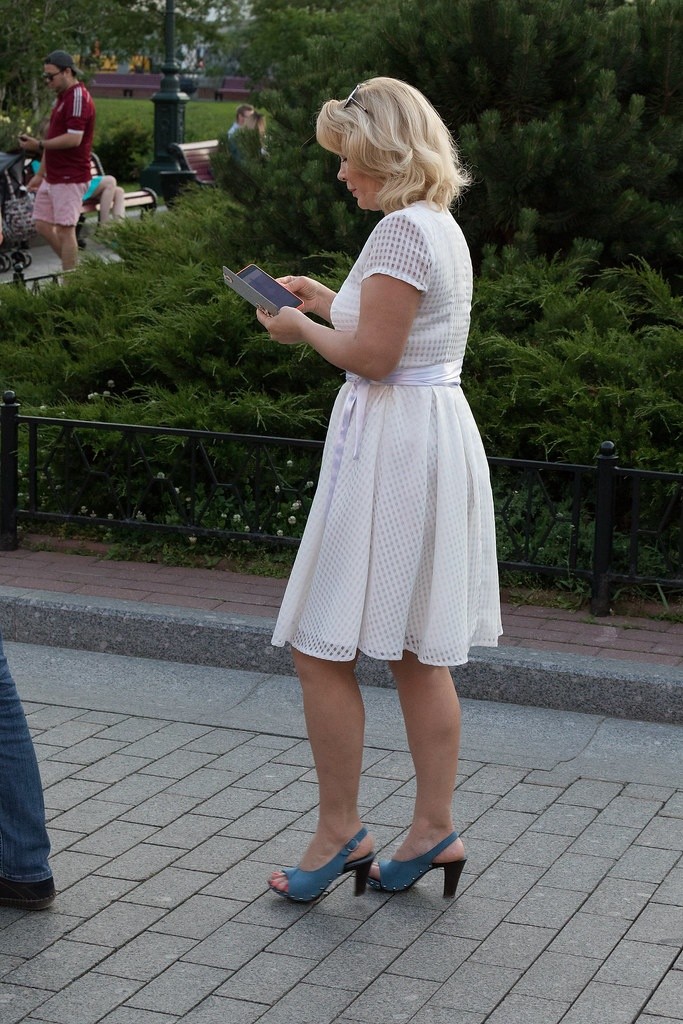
[42,70,63,82]
[344,85,369,114]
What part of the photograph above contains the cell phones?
[236,264,304,311]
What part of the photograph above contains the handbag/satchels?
[4,169,40,242]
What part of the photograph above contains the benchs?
[0,139,222,274]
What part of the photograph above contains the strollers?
[0,151,35,274]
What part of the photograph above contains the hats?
[45,50,84,75]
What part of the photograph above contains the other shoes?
[0,877,57,910]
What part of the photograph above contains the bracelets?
[39,141,44,150]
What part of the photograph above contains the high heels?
[367,831,467,898]
[267,827,375,903]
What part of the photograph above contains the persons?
[32,120,124,226]
[18,50,96,286]
[256,77,503,904]
[0,630,54,912]
[228,104,267,163]
